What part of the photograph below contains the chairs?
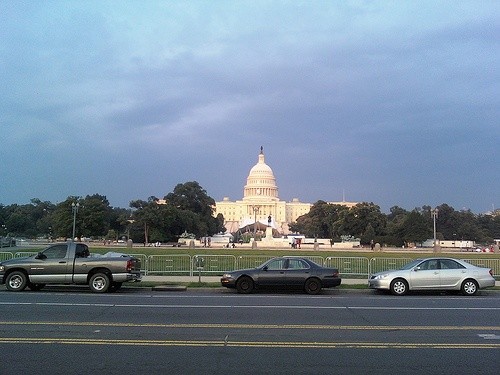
[281,260,287,269]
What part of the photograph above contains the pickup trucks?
[0,240,143,293]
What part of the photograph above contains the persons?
[482,247,489,252]
[330,239,334,248]
[298,239,302,249]
[475,248,481,252]
[294,238,298,249]
[490,246,494,252]
[232,243,236,248]
[370,240,374,250]
[226,243,229,248]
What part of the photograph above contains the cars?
[219,256,343,296]
[0,238,16,247]
[366,256,496,296]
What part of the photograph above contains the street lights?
[429,207,438,249]
[250,203,261,241]
[70,202,81,239]
